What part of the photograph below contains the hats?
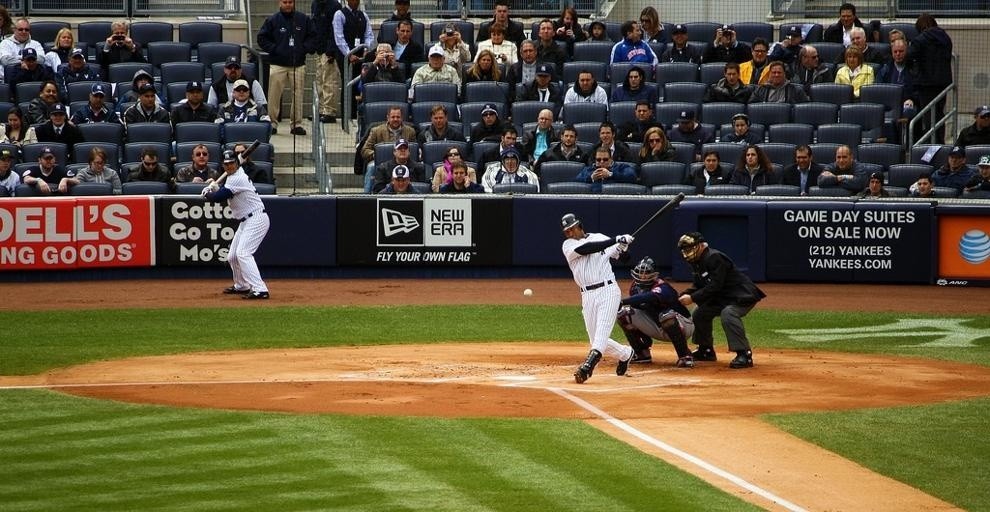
[561,213,580,231]
[223,150,237,163]
[429,46,444,56]
[22,48,37,59]
[536,64,551,76]
[677,109,695,123]
[90,55,249,95]
[670,24,687,33]
[481,104,498,116]
[49,103,66,113]
[392,139,410,179]
[69,48,84,58]
[0,147,54,159]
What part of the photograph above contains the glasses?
[448,153,459,156]
[484,112,494,116]
[144,161,157,165]
[196,152,207,156]
[235,87,249,92]
[596,157,608,162]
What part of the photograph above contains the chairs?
[358,22,988,203]
[0,21,279,197]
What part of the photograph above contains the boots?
[574,350,603,383]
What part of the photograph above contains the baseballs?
[958,228,990,265]
[523,289,531,298]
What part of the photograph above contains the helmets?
[678,232,708,263]
[631,256,657,284]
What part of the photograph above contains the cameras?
[446,28,455,36]
[723,26,732,37]
[384,53,390,60]
[494,52,501,60]
[113,34,125,41]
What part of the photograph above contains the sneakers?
[308,114,336,123]
[730,349,753,368]
[616,348,651,375]
[291,127,306,135]
[677,345,716,367]
[223,286,269,299]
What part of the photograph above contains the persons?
[256,0,311,136]
[678,231,767,369]
[617,256,697,369]
[307,0,342,124]
[202,150,271,300]
[0,6,275,194]
[332,0,374,130]
[561,213,634,384]
[360,1,990,196]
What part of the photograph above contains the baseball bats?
[214,140,262,183]
[631,193,685,234]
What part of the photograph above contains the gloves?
[201,182,220,197]
[608,234,634,260]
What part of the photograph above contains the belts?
[241,209,267,222]
[582,280,611,291]
